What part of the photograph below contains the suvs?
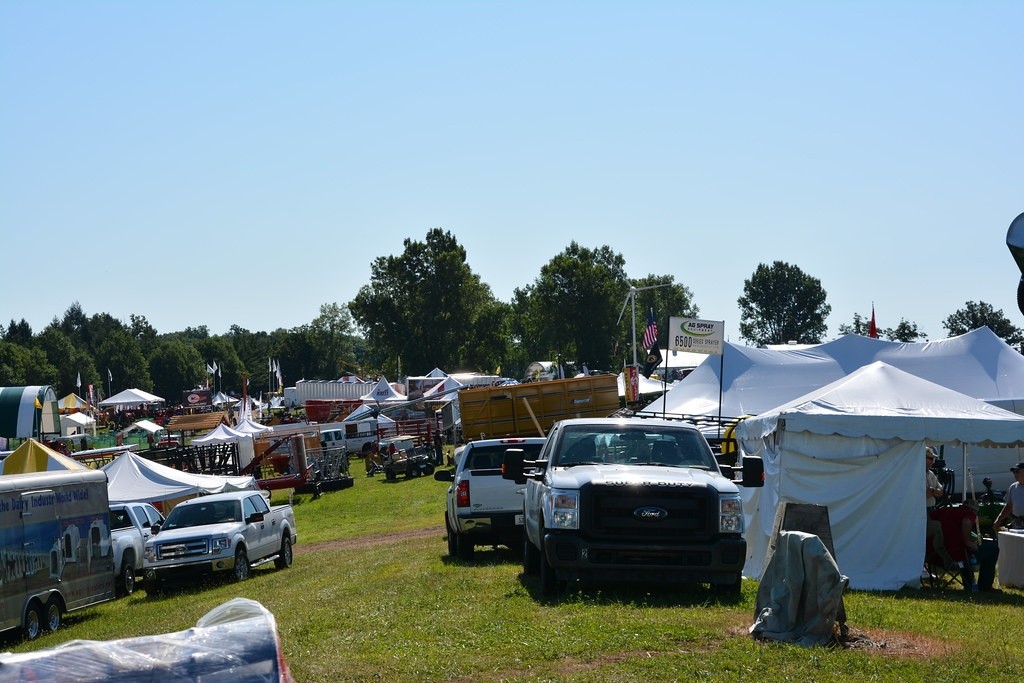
[501,417,766,603]
[434,435,554,561]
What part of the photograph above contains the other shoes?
[979,587,1002,595]
[964,592,973,602]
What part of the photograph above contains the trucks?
[0,468,118,643]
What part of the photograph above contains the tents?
[336,366,519,399]
[617,325,1024,590]
[193,421,274,469]
[523,361,565,382]
[0,385,96,439]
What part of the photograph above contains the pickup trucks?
[109,501,165,595]
[144,489,298,596]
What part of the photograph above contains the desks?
[998,531,1024,588]
[72,444,137,470]
[156,441,178,448]
[163,435,181,441]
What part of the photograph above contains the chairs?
[924,520,980,588]
[473,455,491,467]
[652,440,678,462]
[566,438,596,457]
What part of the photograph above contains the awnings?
[1,440,255,512]
[212,391,264,406]
[100,388,165,416]
[116,420,164,435]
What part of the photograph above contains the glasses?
[1013,469,1024,473]
[926,456,933,461]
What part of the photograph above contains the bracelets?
[931,487,936,493]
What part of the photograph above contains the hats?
[926,446,940,457]
[1010,462,1024,471]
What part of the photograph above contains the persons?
[228,407,234,424]
[979,463,1024,532]
[366,439,398,477]
[44,434,68,454]
[926,447,944,522]
[937,500,1003,598]
[80,436,87,451]
[251,400,308,420]
[110,403,220,432]
[142,431,154,448]
[62,407,69,415]
[321,440,328,457]
[70,431,76,435]
[434,429,445,466]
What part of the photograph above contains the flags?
[642,307,657,350]
[76,371,81,387]
[495,365,501,374]
[207,361,218,374]
[218,364,221,377]
[268,357,283,386]
[870,305,876,339]
[108,368,112,381]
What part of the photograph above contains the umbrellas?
[57,392,96,413]
